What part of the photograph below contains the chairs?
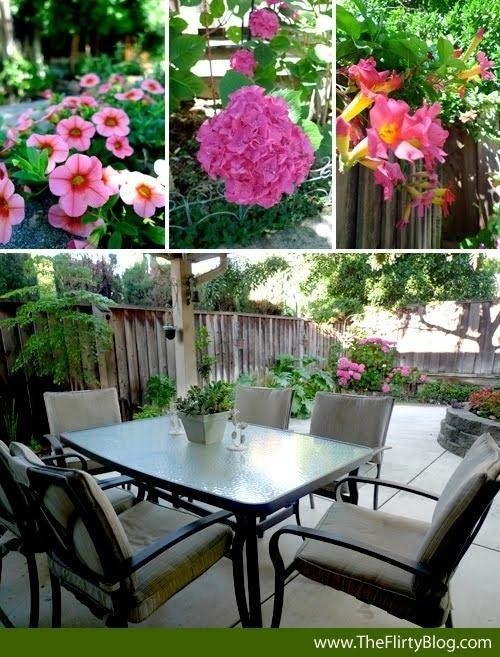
[0,384,500,628]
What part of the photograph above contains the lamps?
[186,273,200,305]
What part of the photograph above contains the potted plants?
[176,379,236,444]
